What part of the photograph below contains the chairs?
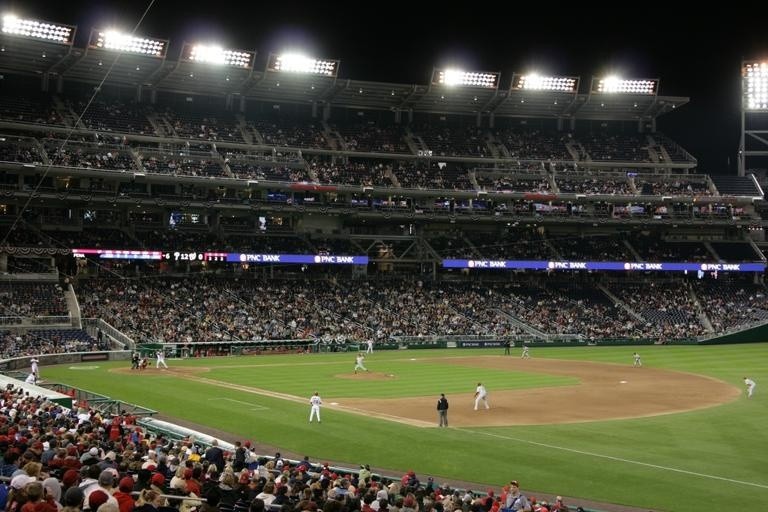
[1,88,768,360]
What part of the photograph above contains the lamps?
[1,12,768,117]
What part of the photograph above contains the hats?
[10,469,38,491]
[151,473,166,486]
[89,490,108,506]
[64,486,85,503]
[291,465,416,501]
[62,470,83,485]
[118,477,134,492]
[32,442,44,452]
[98,470,113,485]
[90,447,98,455]
[240,474,267,487]
[184,470,192,477]
